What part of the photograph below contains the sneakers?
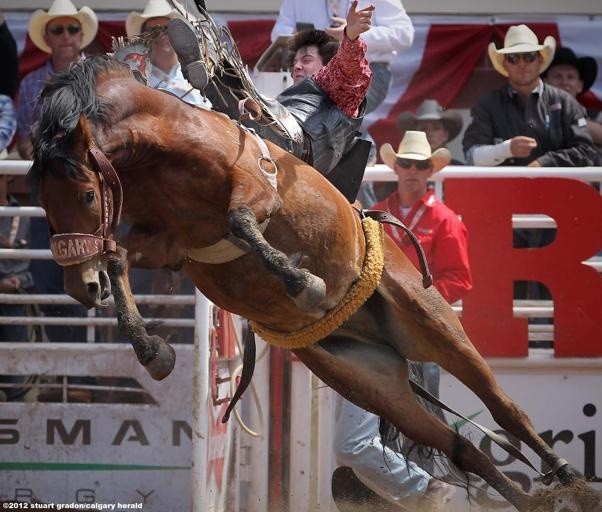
[422,476,467,511]
[167,17,212,90]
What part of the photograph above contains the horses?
[25,53,586,512]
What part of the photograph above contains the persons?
[271,0,415,115]
[398,99,466,165]
[332,130,473,512]
[167,0,375,176]
[463,24,596,349]
[0,200,52,401]
[0,94,18,152]
[16,0,105,402]
[540,48,602,145]
[114,0,214,404]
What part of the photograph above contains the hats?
[28,0,98,54]
[379,131,453,175]
[487,24,557,78]
[125,0,184,41]
[397,99,463,143]
[554,47,597,93]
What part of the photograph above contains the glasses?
[51,23,81,35]
[504,51,537,64]
[394,158,431,170]
